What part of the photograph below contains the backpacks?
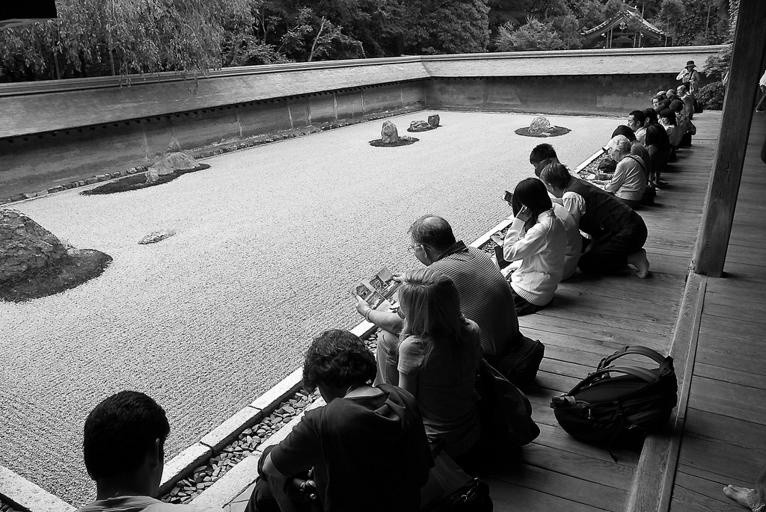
[551,344,678,464]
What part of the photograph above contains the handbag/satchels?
[420,443,495,512]
[480,331,545,386]
[481,358,539,445]
[625,156,656,205]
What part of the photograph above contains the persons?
[584,133,656,210]
[502,176,570,315]
[72,390,225,512]
[755,71,766,165]
[669,100,696,148]
[541,162,650,280]
[644,127,664,192]
[244,326,434,512]
[683,83,695,115]
[642,107,672,164]
[651,94,665,113]
[677,85,695,120]
[524,142,581,205]
[676,61,700,114]
[666,88,686,116]
[611,125,653,178]
[626,110,648,148]
[658,109,684,163]
[397,270,511,458]
[353,214,523,388]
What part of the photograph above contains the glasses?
[407,243,432,255]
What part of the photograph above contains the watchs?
[364,308,375,328]
[365,307,371,322]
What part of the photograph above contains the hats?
[684,61,696,69]
